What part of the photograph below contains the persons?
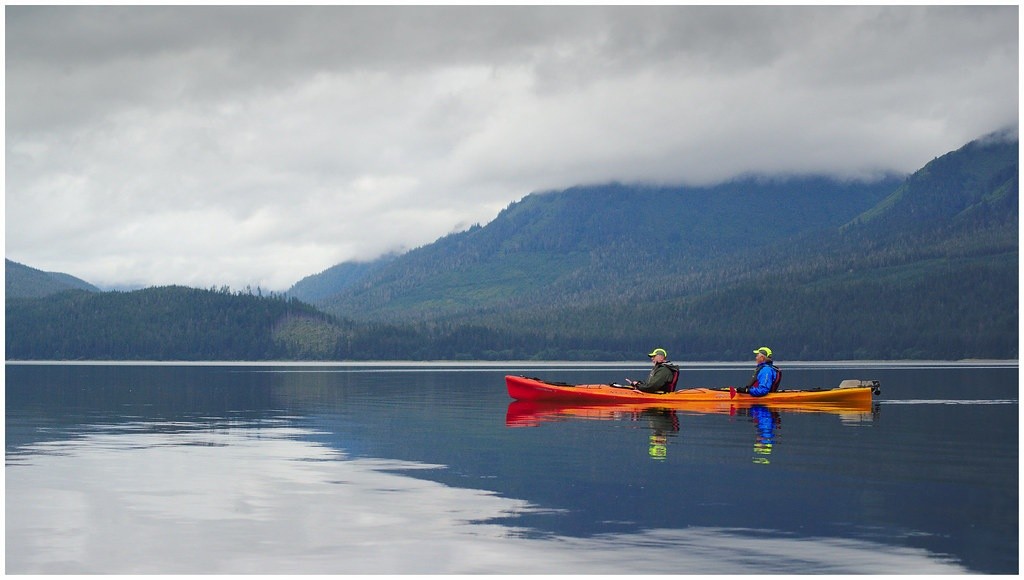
[627,346,680,394]
[736,345,780,397]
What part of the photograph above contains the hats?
[752,347,772,358]
[648,348,667,357]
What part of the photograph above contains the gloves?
[736,387,746,393]
[631,380,641,387]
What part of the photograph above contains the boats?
[504,374,881,401]
